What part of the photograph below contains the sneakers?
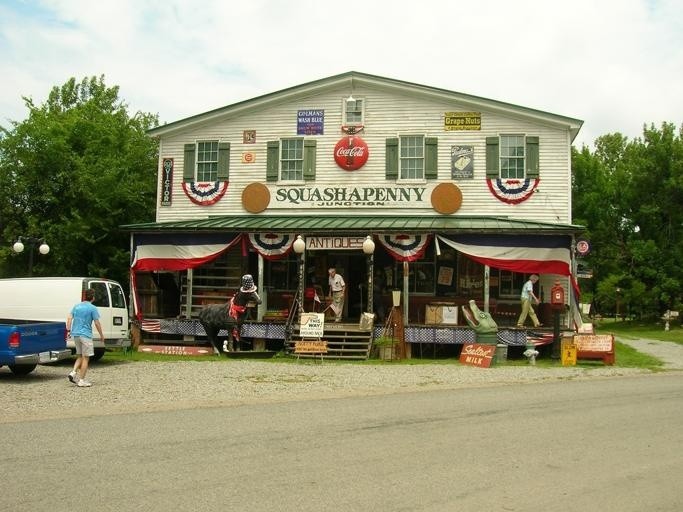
[68,372,77,383]
[77,379,91,386]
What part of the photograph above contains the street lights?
[13,234,52,277]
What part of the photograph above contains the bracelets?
[65,329,71,332]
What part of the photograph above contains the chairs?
[312,285,334,323]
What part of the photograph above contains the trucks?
[0,275,134,365]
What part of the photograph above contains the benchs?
[573,334,615,364]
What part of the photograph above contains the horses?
[198,292,262,356]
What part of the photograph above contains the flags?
[313,293,321,304]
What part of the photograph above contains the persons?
[326,268,347,324]
[516,274,545,328]
[65,288,105,386]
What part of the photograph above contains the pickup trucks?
[0,317,73,377]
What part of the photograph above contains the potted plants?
[373,336,400,360]
